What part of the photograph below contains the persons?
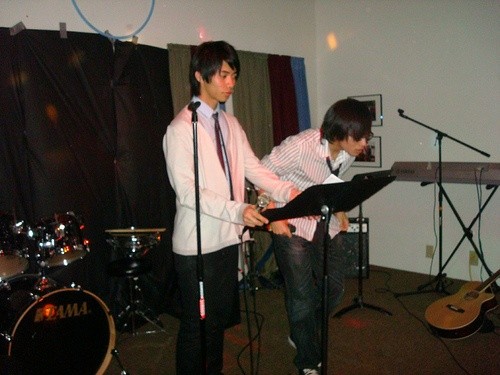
[252,98,374,375]
[162,40,321,375]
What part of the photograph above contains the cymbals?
[105,228,167,237]
[0,250,28,281]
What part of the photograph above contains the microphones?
[242,193,271,235]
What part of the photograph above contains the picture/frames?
[350,136,382,169]
[353,95,382,126]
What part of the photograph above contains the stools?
[106,257,164,329]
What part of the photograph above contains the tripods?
[395,136,452,295]
[332,203,393,319]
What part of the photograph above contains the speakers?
[345,217,369,279]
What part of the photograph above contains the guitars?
[426,269,500,339]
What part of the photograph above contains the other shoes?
[288,336,322,375]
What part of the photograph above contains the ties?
[326,157,342,177]
[211,112,234,202]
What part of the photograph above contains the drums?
[27,214,89,266]
[0,274,116,375]
